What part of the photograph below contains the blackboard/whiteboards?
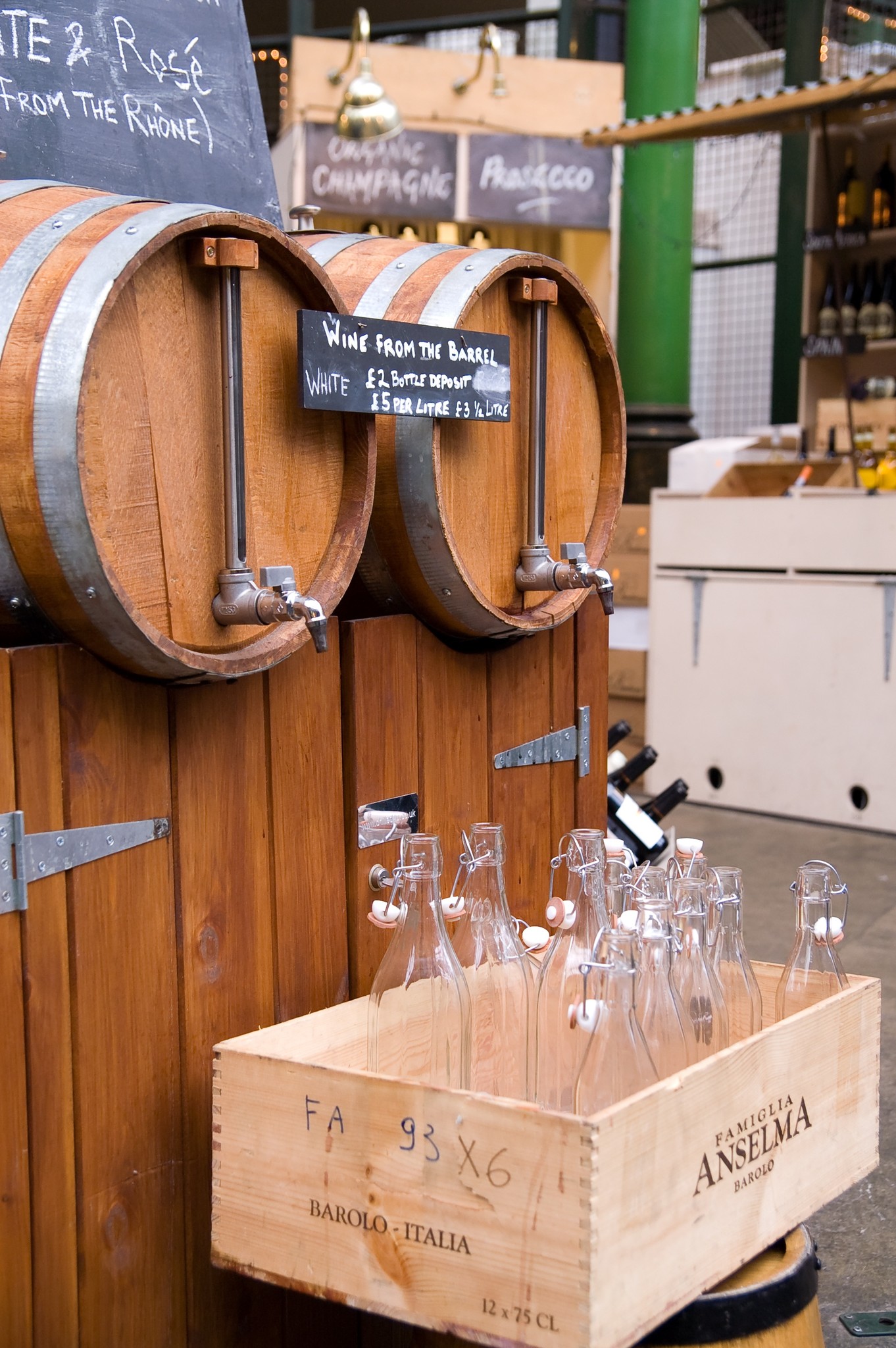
[293,308,513,422]
[1,0,287,235]
[293,111,622,234]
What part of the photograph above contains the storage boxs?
[207,959,884,1348]
[815,396,896,453]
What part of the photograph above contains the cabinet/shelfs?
[798,113,896,444]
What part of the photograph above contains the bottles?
[868,147,896,229]
[852,374,896,398]
[834,144,866,232]
[817,261,841,339]
[823,424,838,458]
[605,781,669,862]
[841,257,896,341]
[774,859,854,1023]
[795,426,809,460]
[781,466,813,497]
[607,744,658,794]
[853,422,896,493]
[607,718,634,751]
[641,776,689,823]
[364,831,473,1092]
[450,820,538,1103]
[535,828,764,1118]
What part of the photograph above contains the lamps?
[329,8,404,139]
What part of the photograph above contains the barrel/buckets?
[634,1219,826,1348]
[1,176,380,689]
[285,230,628,651]
